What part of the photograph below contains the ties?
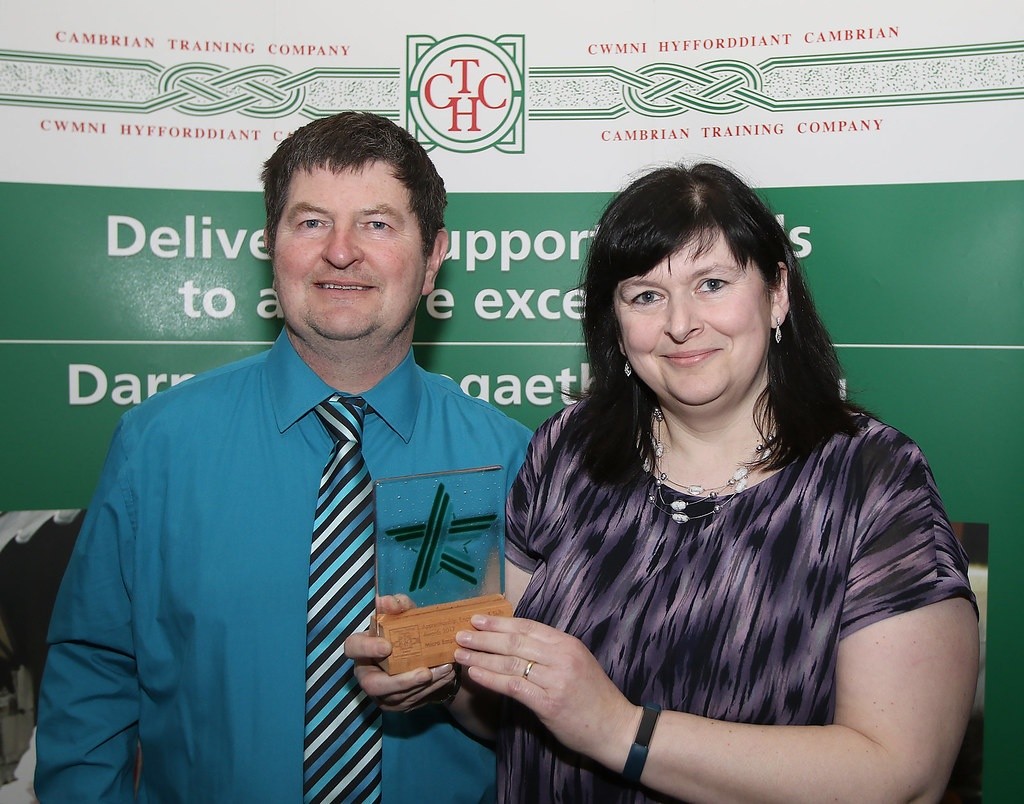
[303,395,382,804]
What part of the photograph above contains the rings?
[523,660,534,678]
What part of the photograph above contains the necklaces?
[647,407,773,523]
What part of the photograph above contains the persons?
[32,112,536,804]
[344,163,981,804]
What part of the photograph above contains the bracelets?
[440,664,461,702]
[622,704,661,784]
